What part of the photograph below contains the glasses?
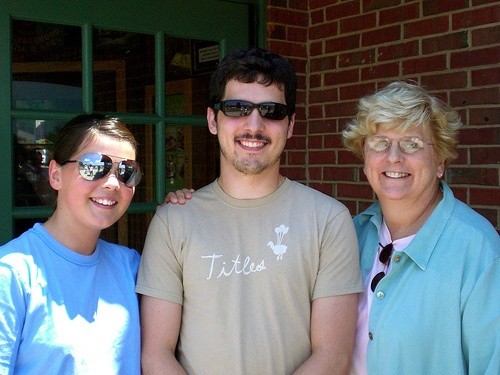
[367,134,436,155]
[371,242,393,292]
[66,151,144,188]
[214,99,293,121]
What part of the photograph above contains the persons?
[0,114,195,375]
[342,81,500,375]
[136,48,364,375]
